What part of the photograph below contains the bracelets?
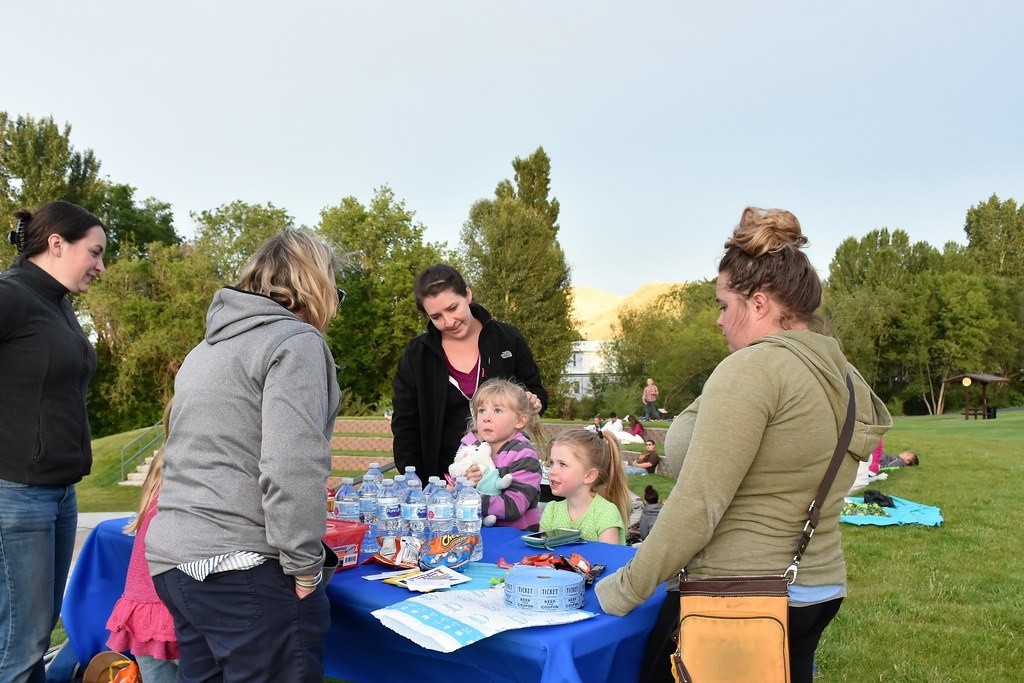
[294,572,322,588]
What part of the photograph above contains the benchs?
[961,412,989,420]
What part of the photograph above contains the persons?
[847,453,873,491]
[627,415,644,439]
[628,484,663,547]
[641,378,660,420]
[106,395,180,683]
[594,207,893,683]
[624,439,659,476]
[445,376,542,533]
[601,412,623,432]
[390,265,549,492]
[0,199,106,683]
[539,429,630,547]
[868,436,883,477]
[593,416,603,430]
[878,451,919,469]
[144,231,346,683]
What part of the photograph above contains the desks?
[42,514,668,683]
[969,408,983,420]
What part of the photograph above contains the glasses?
[646,444,652,446]
[335,285,346,307]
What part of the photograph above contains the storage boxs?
[322,518,369,574]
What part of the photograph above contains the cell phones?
[521,528,581,543]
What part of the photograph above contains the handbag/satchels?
[670,575,791,683]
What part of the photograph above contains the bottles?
[333,463,483,563]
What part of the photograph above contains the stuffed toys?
[448,441,513,527]
